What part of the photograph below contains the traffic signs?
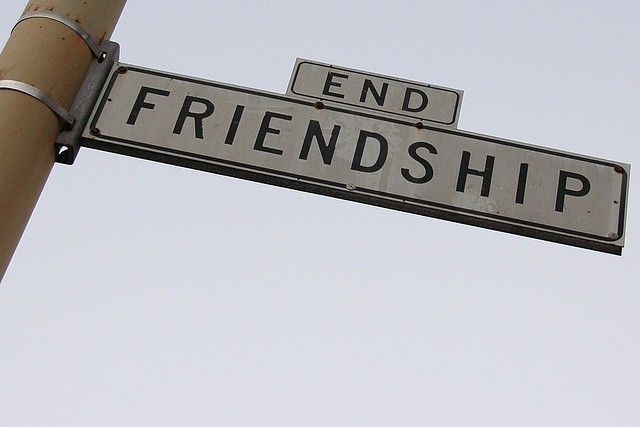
[53,34,633,257]
